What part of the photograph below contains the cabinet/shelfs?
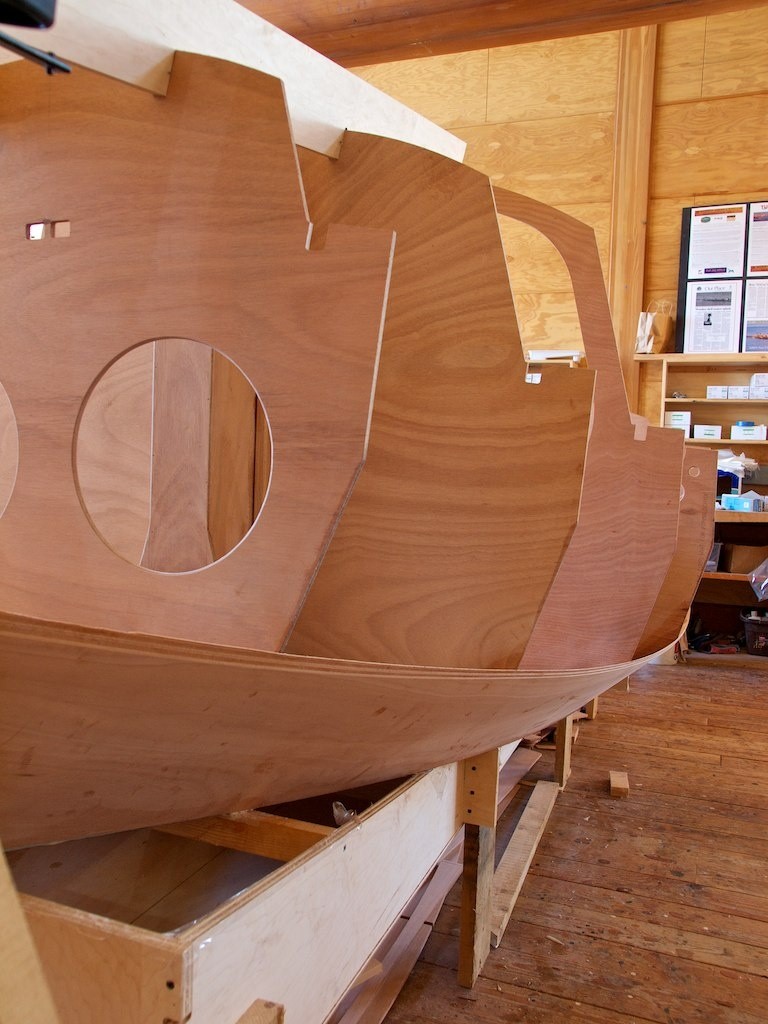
[634,353,768,605]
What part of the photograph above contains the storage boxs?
[694,425,722,439]
[723,543,768,574]
[730,425,767,441]
[706,385,728,400]
[722,494,764,512]
[750,373,768,386]
[664,424,690,438]
[749,386,768,400]
[664,411,692,425]
[728,386,749,399]
